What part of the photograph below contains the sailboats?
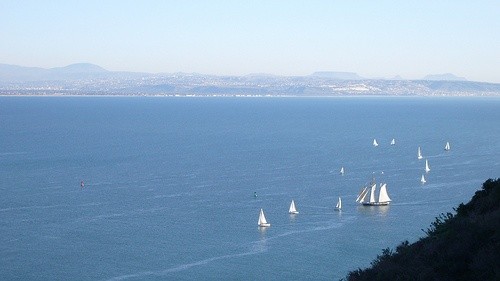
[289,200,300,214]
[335,197,342,209]
[373,139,378,146]
[419,174,426,183]
[425,160,430,173]
[355,183,393,205]
[417,144,423,160]
[258,208,271,227]
[390,138,395,145]
[444,141,451,150]
[338,165,346,175]
